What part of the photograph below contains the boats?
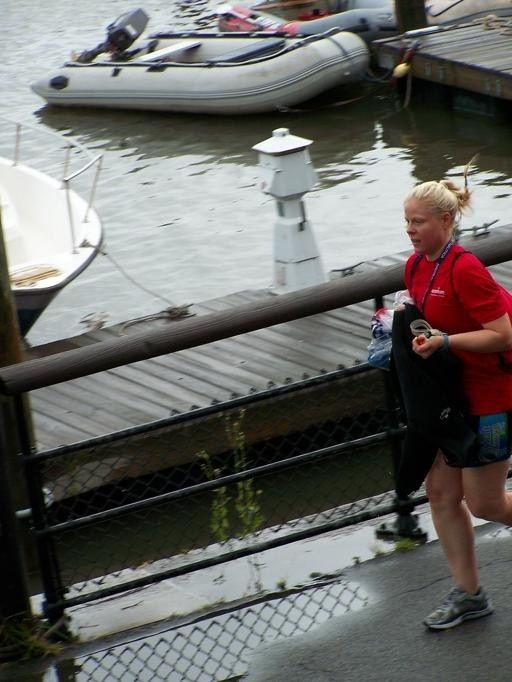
[216,0,512,33]
[27,7,371,115]
[0,115,106,343]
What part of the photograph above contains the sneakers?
[422,585,495,629]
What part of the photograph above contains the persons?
[403,154,512,630]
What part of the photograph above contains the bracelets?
[443,334,449,348]
